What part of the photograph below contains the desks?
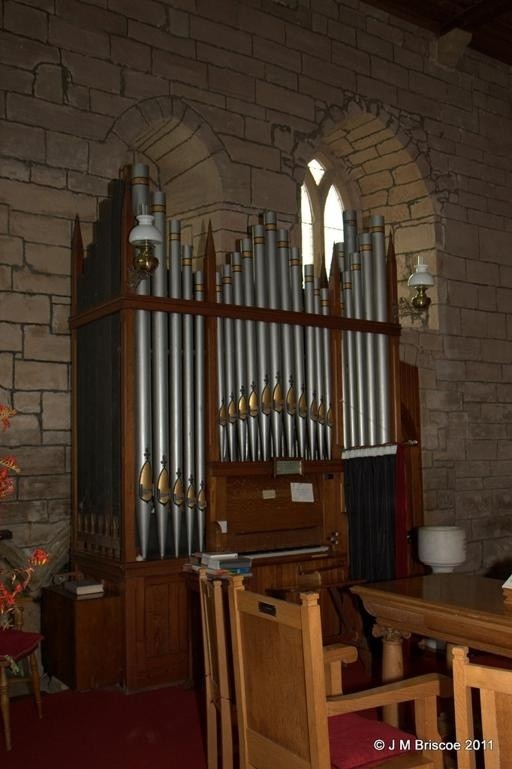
[350,572,512,729]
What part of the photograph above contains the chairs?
[198,567,238,769]
[452,645,512,769]
[226,575,453,769]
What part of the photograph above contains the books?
[67,578,105,595]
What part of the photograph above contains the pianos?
[237,544,328,560]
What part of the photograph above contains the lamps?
[126,204,164,291]
[408,256,435,311]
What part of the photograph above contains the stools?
[0,631,43,752]
